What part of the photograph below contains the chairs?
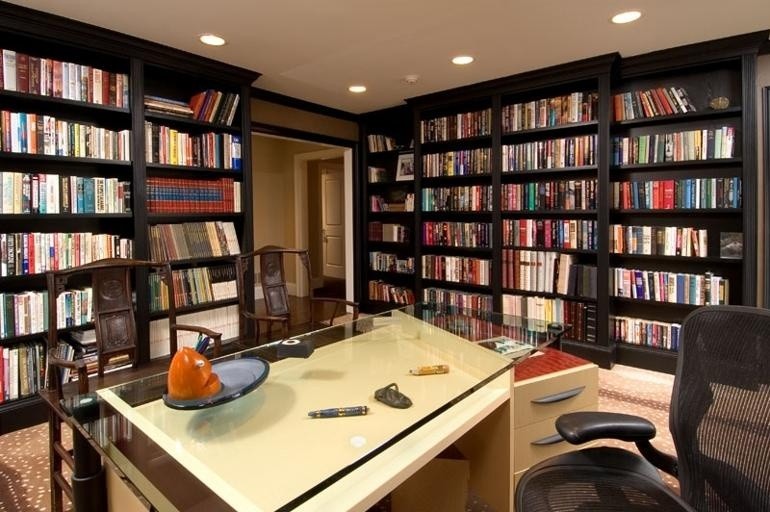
[508,303,769,512]
[231,238,365,362]
[35,252,234,510]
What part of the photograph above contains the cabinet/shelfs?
[429,309,608,510]
[598,26,767,396]
[352,102,425,319]
[401,49,627,373]
[1,0,267,441]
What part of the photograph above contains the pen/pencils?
[307,406,367,419]
[408,365,449,376]
[195,332,210,354]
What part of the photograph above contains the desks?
[61,302,582,512]
